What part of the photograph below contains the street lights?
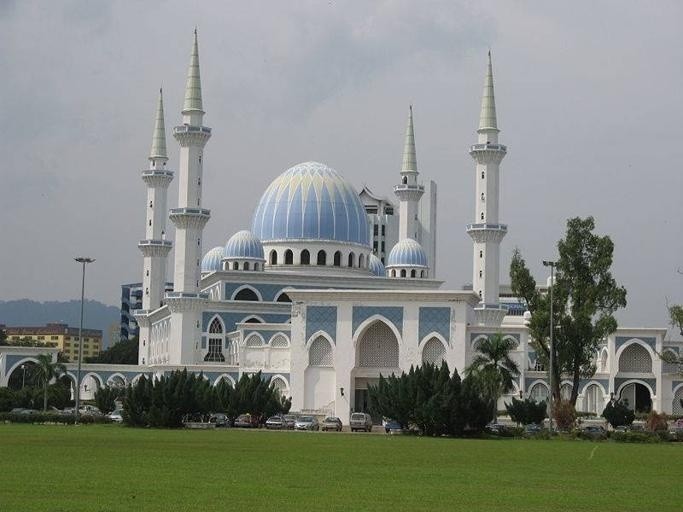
[543,260,559,433]
[74,257,97,425]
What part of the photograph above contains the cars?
[72,404,99,416]
[487,424,562,435]
[579,424,680,441]
[215,412,409,433]
[104,408,125,424]
[8,408,40,416]
